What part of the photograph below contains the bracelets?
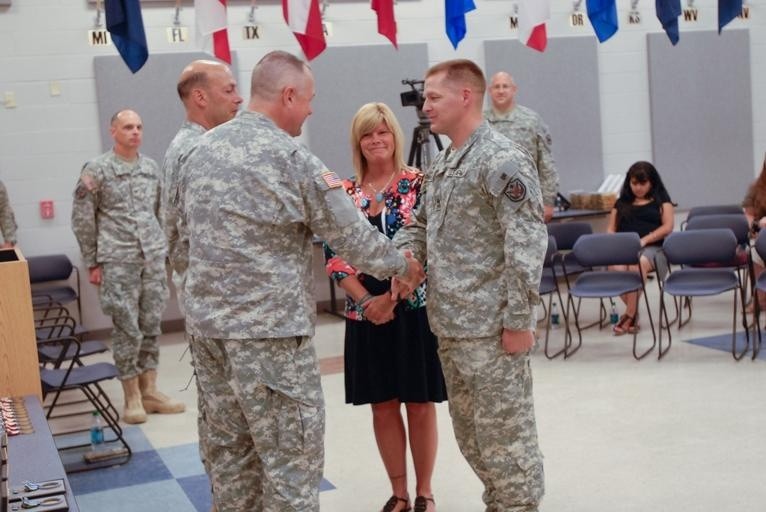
[649,230,659,242]
[358,291,373,307]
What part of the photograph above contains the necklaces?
[366,169,396,203]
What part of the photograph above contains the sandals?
[414,495,435,511]
[628,314,640,333]
[382,492,412,512]
[614,313,633,334]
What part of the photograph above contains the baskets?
[569,193,592,209]
[592,194,616,210]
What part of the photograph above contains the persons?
[70,111,186,424]
[741,153,766,314]
[390,56,548,512]
[605,157,676,335]
[159,58,244,512]
[486,72,560,224]
[1,177,17,251]
[320,101,453,511]
[170,52,425,512]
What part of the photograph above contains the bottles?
[89,411,105,448]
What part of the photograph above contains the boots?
[122,376,146,424]
[139,370,186,414]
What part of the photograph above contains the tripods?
[407,118,446,170]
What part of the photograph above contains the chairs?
[26,255,132,475]
[530,203,762,362]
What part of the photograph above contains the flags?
[370,1,398,51]
[104,0,149,75]
[445,1,479,53]
[717,0,742,37]
[584,0,619,43]
[655,1,682,47]
[516,0,550,52]
[282,0,326,61]
[193,0,232,65]
